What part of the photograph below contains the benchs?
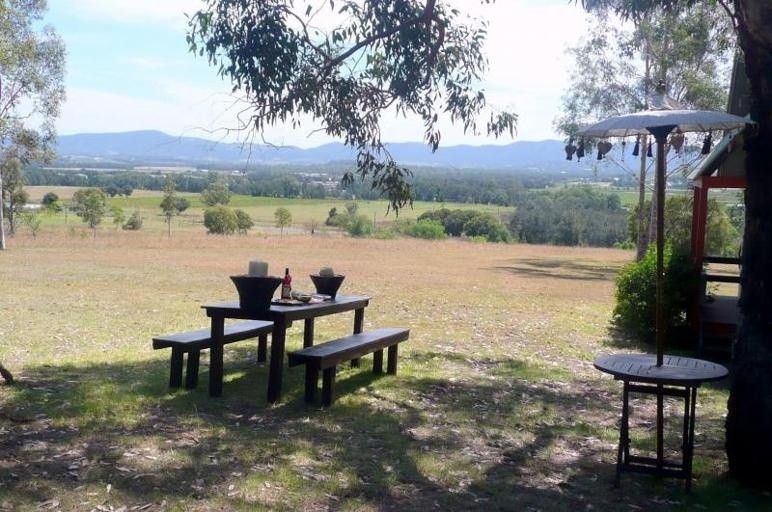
[288,328,410,408]
[153,320,292,387]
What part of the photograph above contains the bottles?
[282,267,292,300]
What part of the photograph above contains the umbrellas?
[571,100,756,479]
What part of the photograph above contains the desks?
[593,352,731,494]
[201,292,371,403]
